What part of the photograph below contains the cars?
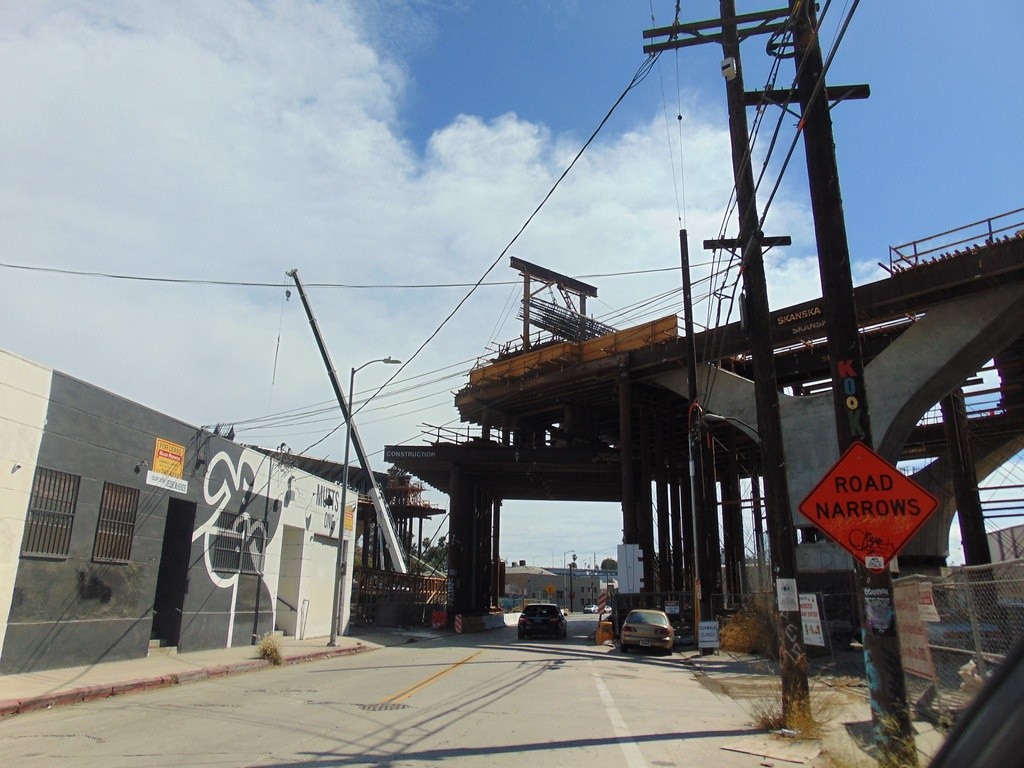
[620,609,674,654]
[517,603,569,641]
[599,604,612,615]
[582,605,599,615]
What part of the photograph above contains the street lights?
[325,357,401,649]
[564,549,576,613]
